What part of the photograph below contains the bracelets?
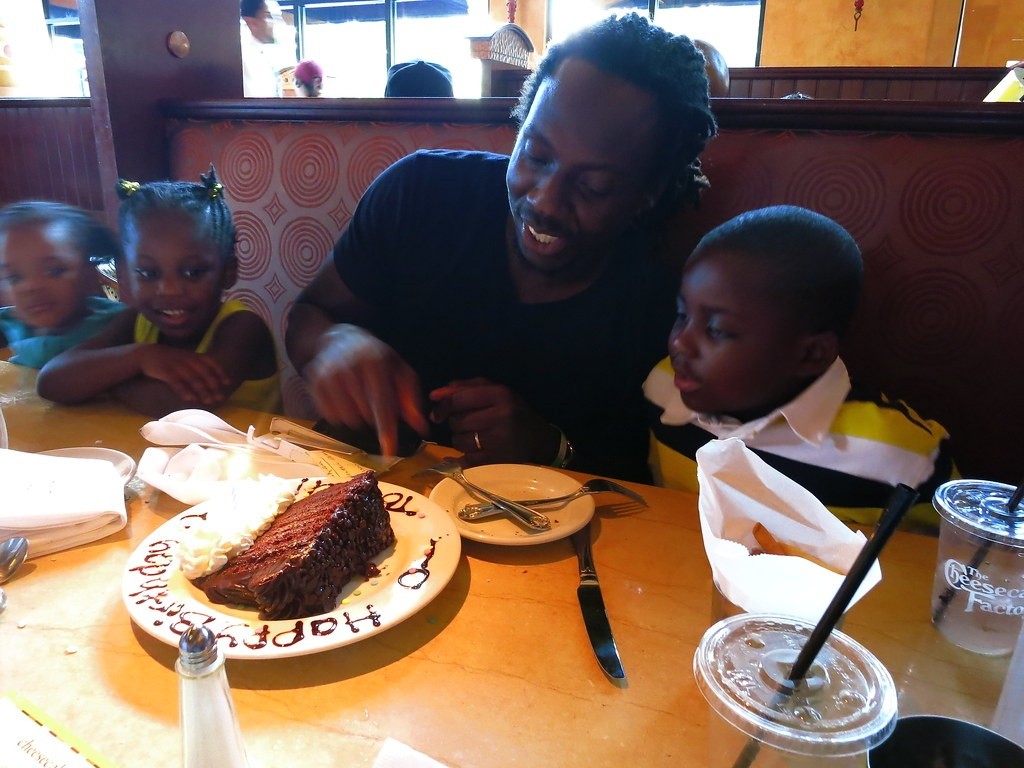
[550,423,575,469]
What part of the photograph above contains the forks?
[410,461,549,531]
[457,477,647,520]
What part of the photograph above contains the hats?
[294,60,322,87]
[384,60,454,98]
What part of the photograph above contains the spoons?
[0,536,30,586]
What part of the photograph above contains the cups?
[693,613,900,768]
[930,480,1023,660]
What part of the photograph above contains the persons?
[295,60,323,96]
[284,12,718,486]
[0,202,128,370]
[383,58,454,97]
[240,0,285,97]
[37,163,282,420]
[642,205,963,537]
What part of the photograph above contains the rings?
[473,432,481,451]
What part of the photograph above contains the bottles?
[175,626,255,766]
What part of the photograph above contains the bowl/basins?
[865,716,1023,768]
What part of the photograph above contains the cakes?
[178,469,398,622]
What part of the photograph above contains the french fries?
[749,523,846,574]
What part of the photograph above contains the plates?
[119,476,461,660]
[429,464,596,545]
[34,447,137,486]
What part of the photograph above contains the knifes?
[570,524,627,680]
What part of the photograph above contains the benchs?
[162,95,1024,534]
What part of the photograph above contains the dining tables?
[12,363,1024,768]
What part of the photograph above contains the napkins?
[0,409,315,559]
